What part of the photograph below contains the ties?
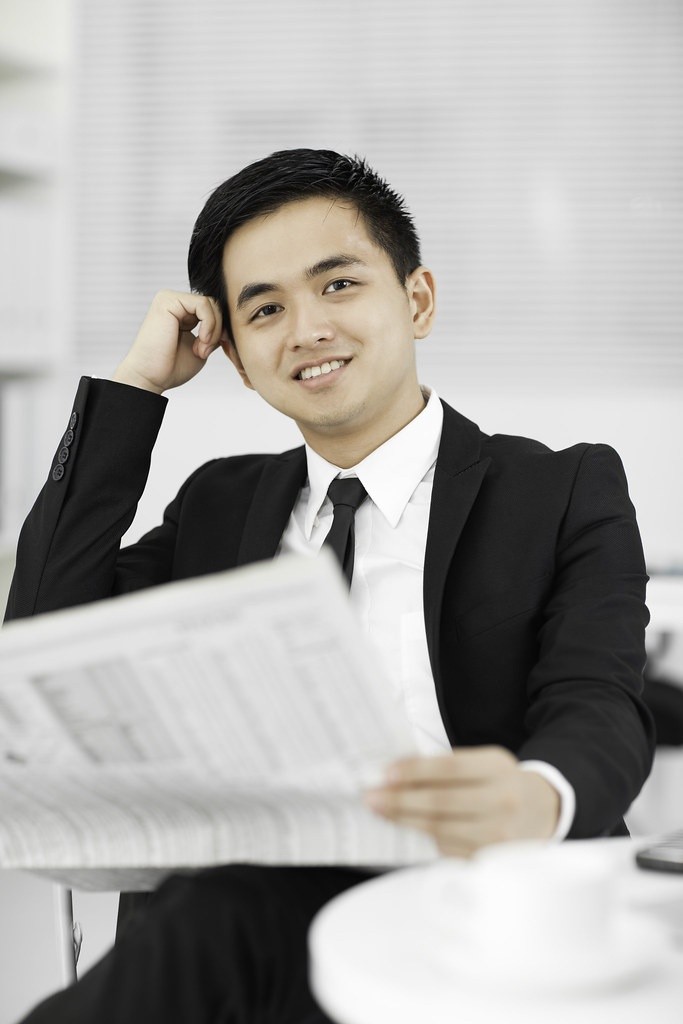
[319,478,368,595]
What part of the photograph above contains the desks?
[307,837,683,1024]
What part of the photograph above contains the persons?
[2,149,655,1024]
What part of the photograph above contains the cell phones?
[633,829,683,875]
